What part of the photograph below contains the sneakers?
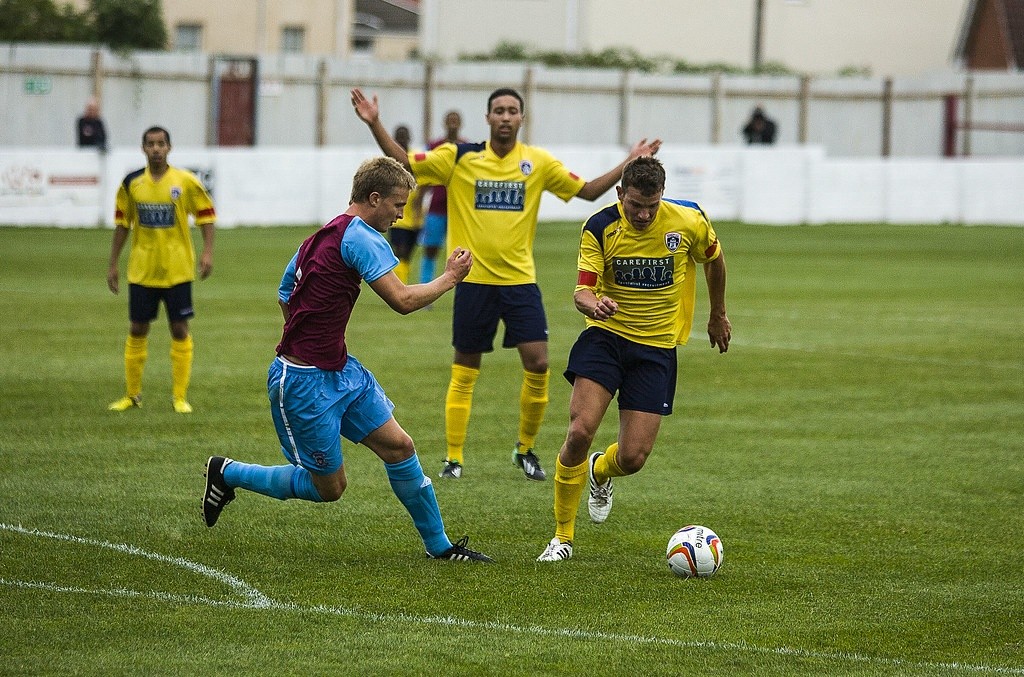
[536,538,572,562]
[426,535,495,563]
[588,452,612,523]
[200,455,236,527]
[109,397,142,412]
[511,446,547,480]
[439,458,462,479]
[172,397,192,414]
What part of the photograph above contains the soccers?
[665,524,724,577]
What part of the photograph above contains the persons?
[744,108,775,145]
[76,98,106,152]
[418,113,467,310]
[107,127,217,415]
[388,127,423,285]
[350,88,661,482]
[537,156,732,563]
[200,158,495,564]
